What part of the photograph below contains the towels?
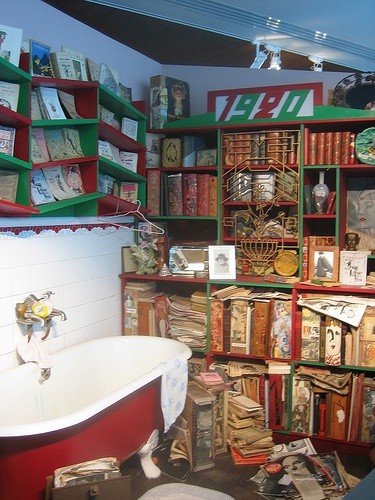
[153,353,189,433]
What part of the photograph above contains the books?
[0,24,138,206]
[123,281,375,500]
[222,128,361,165]
[145,133,218,277]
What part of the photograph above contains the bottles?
[311,172,330,214]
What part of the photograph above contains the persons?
[282,456,327,493]
[316,252,332,277]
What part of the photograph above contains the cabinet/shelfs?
[0,56,375,467]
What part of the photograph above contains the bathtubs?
[0,335,194,500]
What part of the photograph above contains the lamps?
[309,55,323,72]
[266,45,281,70]
[250,41,267,69]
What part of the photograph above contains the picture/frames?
[309,246,340,282]
[208,245,236,279]
[339,251,366,285]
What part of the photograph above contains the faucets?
[41,291,67,322]
[14,291,46,328]
